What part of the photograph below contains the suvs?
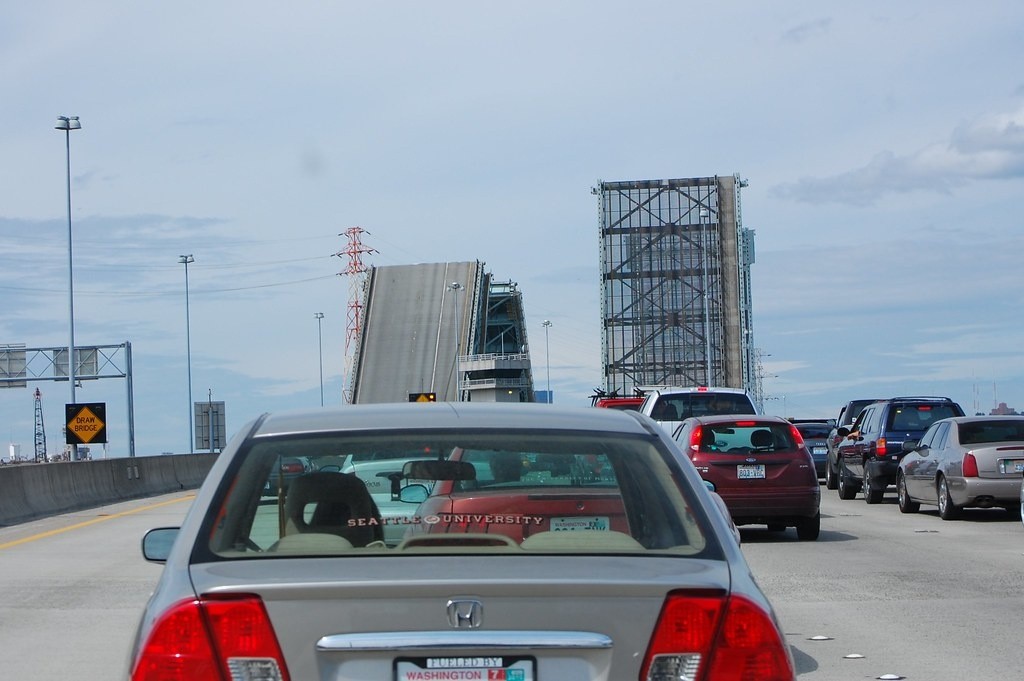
[583,386,774,479]
[785,396,970,505]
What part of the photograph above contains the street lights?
[542,318,552,405]
[313,313,325,406]
[446,282,465,400]
[54,112,82,461]
[178,252,193,454]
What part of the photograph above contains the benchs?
[266,529,647,551]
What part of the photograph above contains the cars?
[316,446,530,547]
[668,415,820,541]
[126,403,796,681]
[896,415,1024,519]
[258,455,349,505]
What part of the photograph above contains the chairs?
[748,430,773,454]
[702,428,715,452]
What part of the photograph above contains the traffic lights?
[428,392,437,403]
